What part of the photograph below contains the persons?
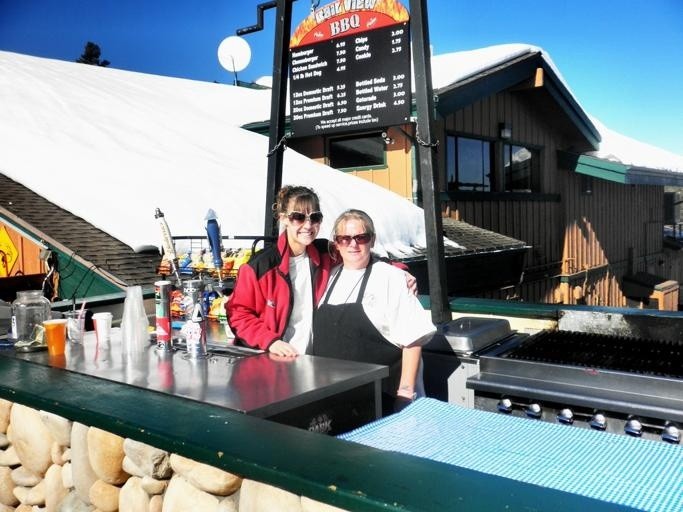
[309,207,438,438]
[225,349,329,412]
[222,184,420,358]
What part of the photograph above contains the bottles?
[11,290,52,353]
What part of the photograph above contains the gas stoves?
[466,328,683,448]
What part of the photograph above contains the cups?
[117,286,149,353]
[41,310,113,356]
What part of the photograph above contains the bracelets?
[396,388,419,399]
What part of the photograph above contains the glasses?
[333,232,374,248]
[284,211,324,225]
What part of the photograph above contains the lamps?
[499,119,512,140]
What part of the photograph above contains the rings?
[412,278,415,282]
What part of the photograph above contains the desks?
[13,326,390,436]
[337,397,683,512]
